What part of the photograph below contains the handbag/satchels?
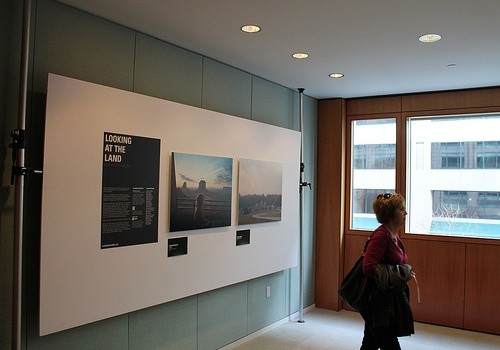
[368,289,414,340]
[339,229,389,311]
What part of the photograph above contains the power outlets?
[266,286,272,298]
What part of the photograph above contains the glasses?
[384,194,392,200]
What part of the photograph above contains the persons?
[360,193,416,350]
